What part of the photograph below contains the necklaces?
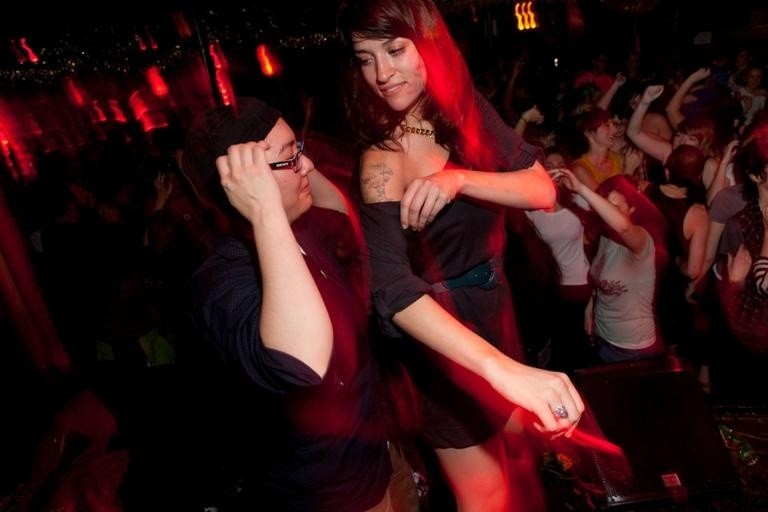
[399,124,435,139]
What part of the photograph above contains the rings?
[553,405,569,421]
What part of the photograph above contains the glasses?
[269,140,304,173]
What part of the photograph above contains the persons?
[298,88,315,144]
[37,134,207,341]
[186,96,392,511]
[1,290,130,512]
[510,48,767,394]
[348,1,587,511]
